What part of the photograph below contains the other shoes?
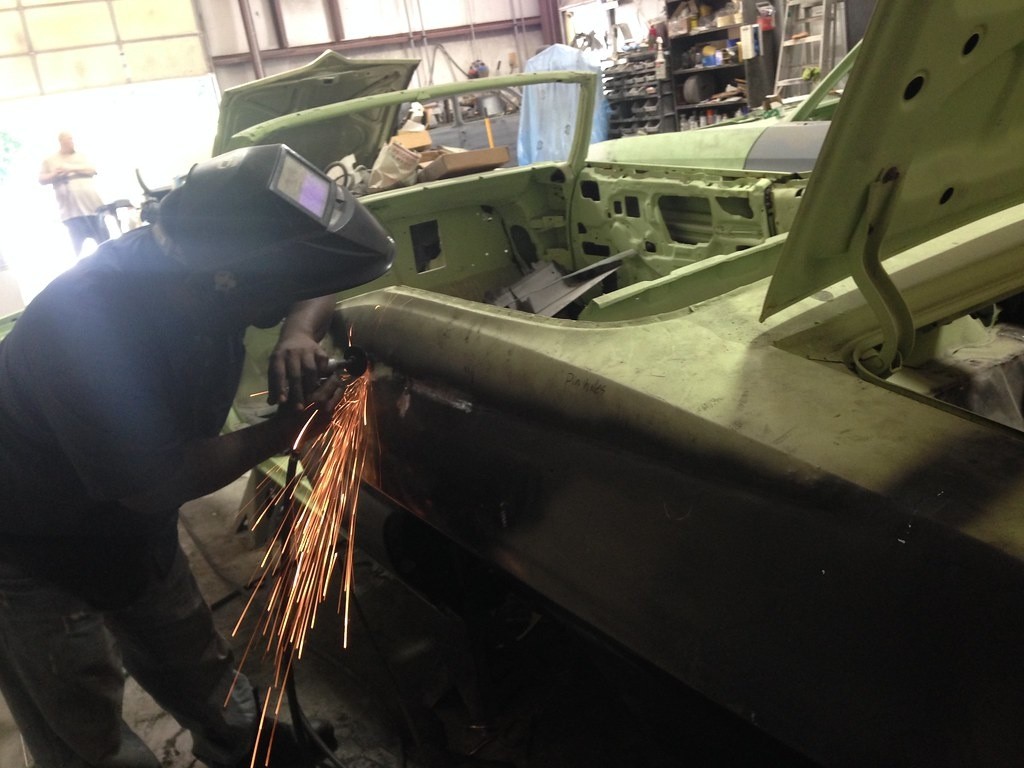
[236,715,334,768]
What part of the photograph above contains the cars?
[211,0,1024,768]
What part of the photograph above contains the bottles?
[656,37,666,80]
[647,25,656,50]
[680,110,728,131]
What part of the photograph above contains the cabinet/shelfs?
[670,22,775,131]
[602,48,675,140]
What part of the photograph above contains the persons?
[39,132,111,258]
[0,142,396,768]
